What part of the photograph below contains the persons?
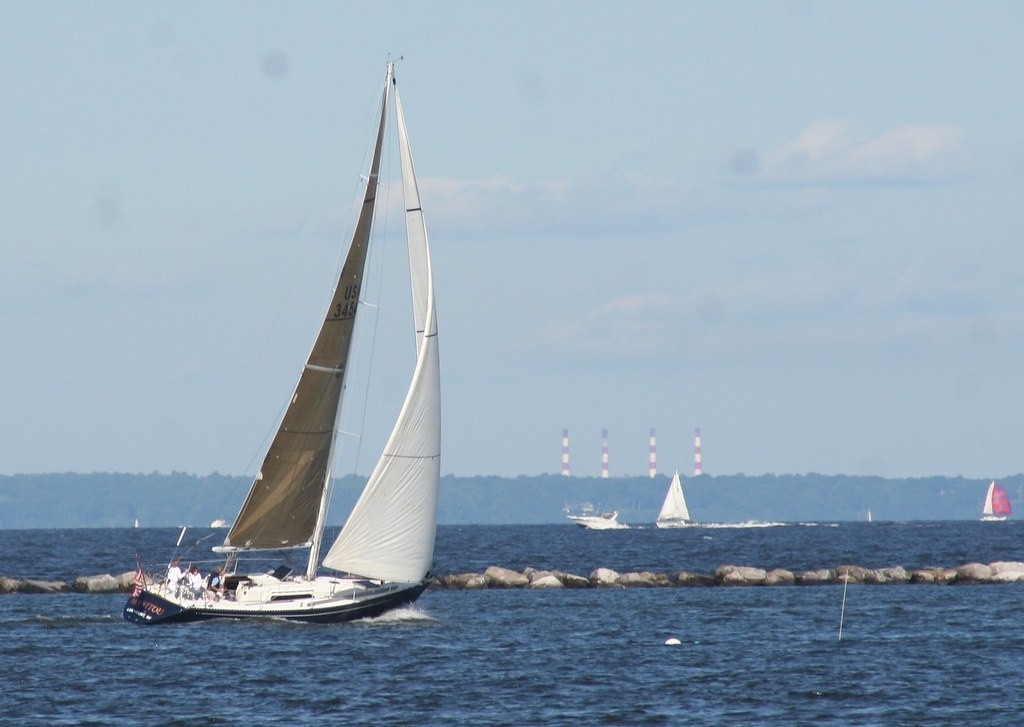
[167,560,225,601]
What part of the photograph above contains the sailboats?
[979,479,1013,522]
[123,50,441,629]
[656,469,698,529]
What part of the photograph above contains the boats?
[566,501,631,530]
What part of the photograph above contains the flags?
[131,561,145,598]
